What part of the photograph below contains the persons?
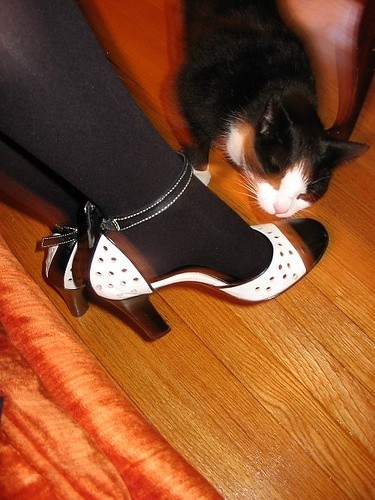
[2,2,329,341]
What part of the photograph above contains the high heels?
[83,151,329,342]
[41,223,92,317]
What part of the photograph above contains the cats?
[174,0,371,220]
